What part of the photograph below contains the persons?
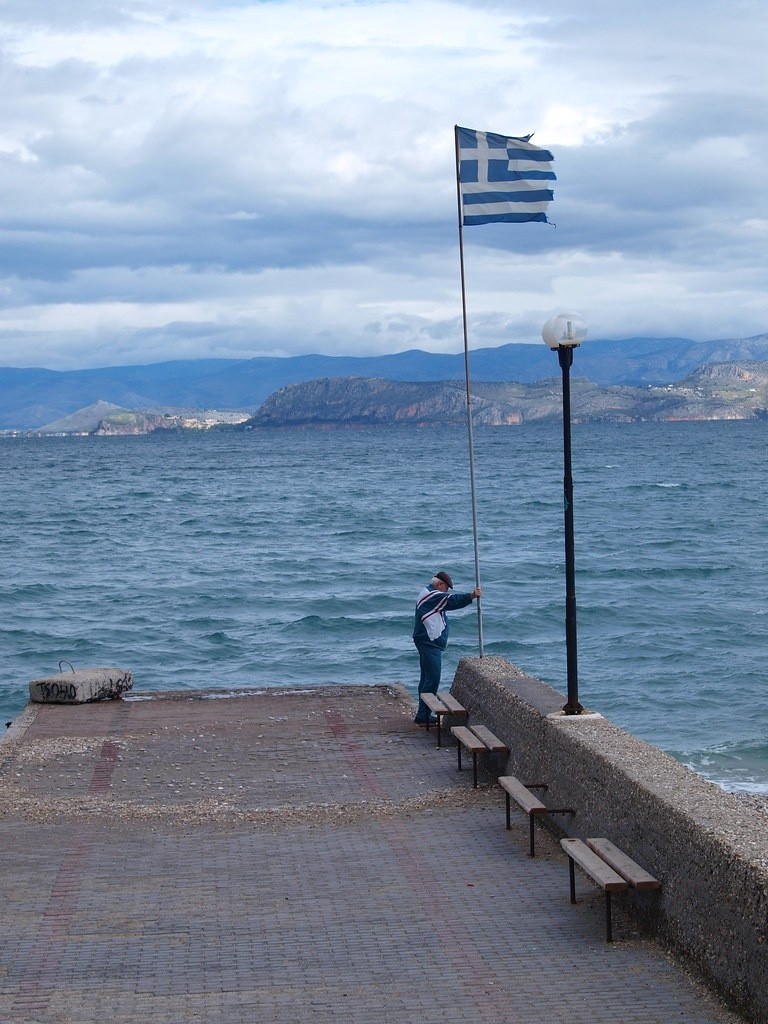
[410,572,481,723]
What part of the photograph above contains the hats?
[437,572,454,589]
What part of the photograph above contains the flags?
[456,125,555,228]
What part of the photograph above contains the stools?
[497,775,576,858]
[420,691,469,747]
[451,724,507,789]
[560,838,660,943]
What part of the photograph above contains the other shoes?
[415,715,439,727]
